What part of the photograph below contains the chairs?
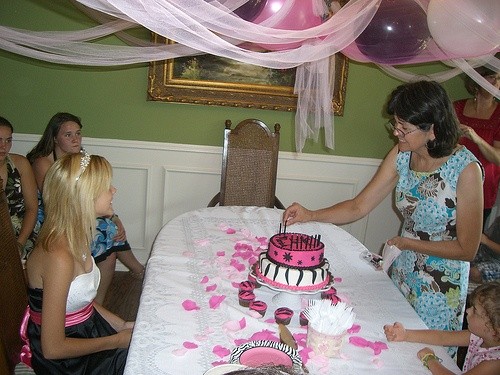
[207,118,286,210]
[0,178,42,375]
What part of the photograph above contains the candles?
[290,233,321,250]
[279,222,282,234]
[284,219,288,233]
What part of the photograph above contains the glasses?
[384,117,421,140]
[484,76,500,85]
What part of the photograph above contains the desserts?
[274,307,294,325]
[239,281,255,293]
[300,311,308,326]
[238,290,256,307]
[325,294,341,306]
[249,301,268,318]
[321,286,337,299]
[248,273,262,288]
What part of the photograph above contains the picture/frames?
[146,32,350,116]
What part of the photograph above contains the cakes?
[255,232,330,291]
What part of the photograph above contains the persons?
[468,215,500,294]
[282,78,485,364]
[451,52,500,233]
[26,112,145,306]
[0,117,44,275]
[19,150,135,375]
[383,281,500,375]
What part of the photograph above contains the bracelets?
[422,354,437,368]
[17,242,24,252]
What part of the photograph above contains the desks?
[121,205,461,375]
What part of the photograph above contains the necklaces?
[82,253,87,261]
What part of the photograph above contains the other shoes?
[128,264,145,280]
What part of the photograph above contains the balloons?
[203,0,499,64]
